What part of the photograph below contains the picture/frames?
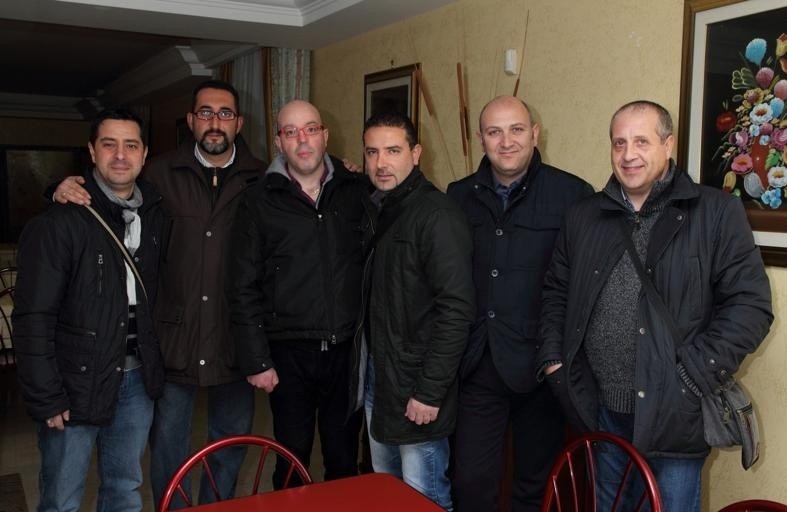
[1,143,86,242]
[361,64,422,174]
[676,0,787,269]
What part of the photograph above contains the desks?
[166,474,447,512]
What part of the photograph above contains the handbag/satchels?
[700,375,763,470]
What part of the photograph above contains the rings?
[47,418,52,425]
[61,192,66,198]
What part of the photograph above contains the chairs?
[159,434,312,511]
[714,499,787,512]
[541,433,663,512]
[0,266,18,422]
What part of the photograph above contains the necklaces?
[305,185,320,201]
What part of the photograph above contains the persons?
[231,99,374,491]
[340,109,476,511]
[44,80,364,512]
[14,107,170,510]
[446,96,596,509]
[536,100,775,512]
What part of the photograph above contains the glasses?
[192,108,239,122]
[278,123,324,139]
